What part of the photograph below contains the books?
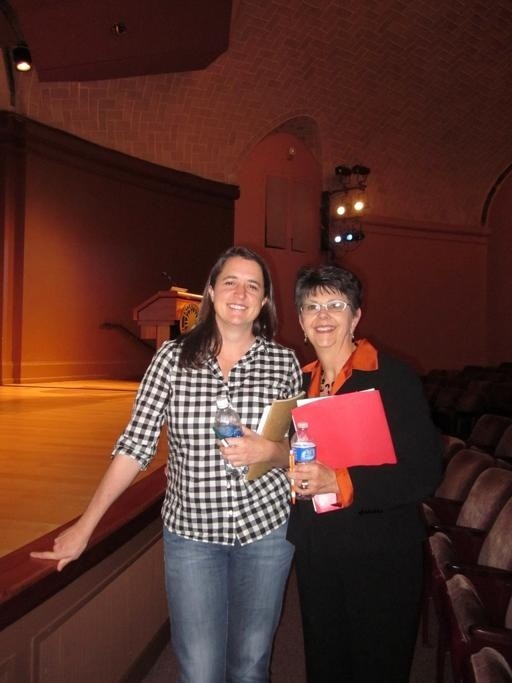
[248,391,303,481]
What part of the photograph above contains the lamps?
[7,36,32,72]
[326,161,371,246]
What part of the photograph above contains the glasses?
[300,300,352,315]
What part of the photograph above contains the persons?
[29,244,301,682]
[286,259,446,682]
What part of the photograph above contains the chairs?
[417,365,511,683]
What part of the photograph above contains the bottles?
[290,422,316,500]
[213,396,248,475]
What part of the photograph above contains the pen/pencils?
[212,426,246,475]
[289,449,297,506]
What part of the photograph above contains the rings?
[301,478,308,489]
